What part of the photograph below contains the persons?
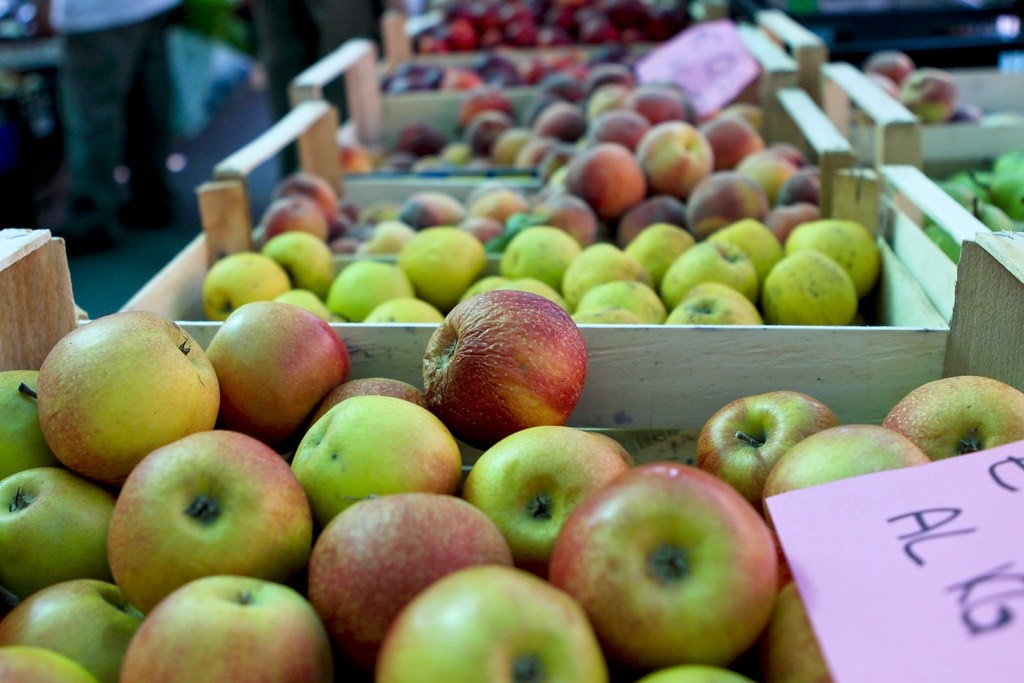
[240,0,322,178]
[46,0,186,256]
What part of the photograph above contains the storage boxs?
[0,0,1024,485]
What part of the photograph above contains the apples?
[199,0,957,331]
[0,289,1023,682]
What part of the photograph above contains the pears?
[920,143,1024,264]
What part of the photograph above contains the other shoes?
[58,228,111,257]
[123,201,176,229]
